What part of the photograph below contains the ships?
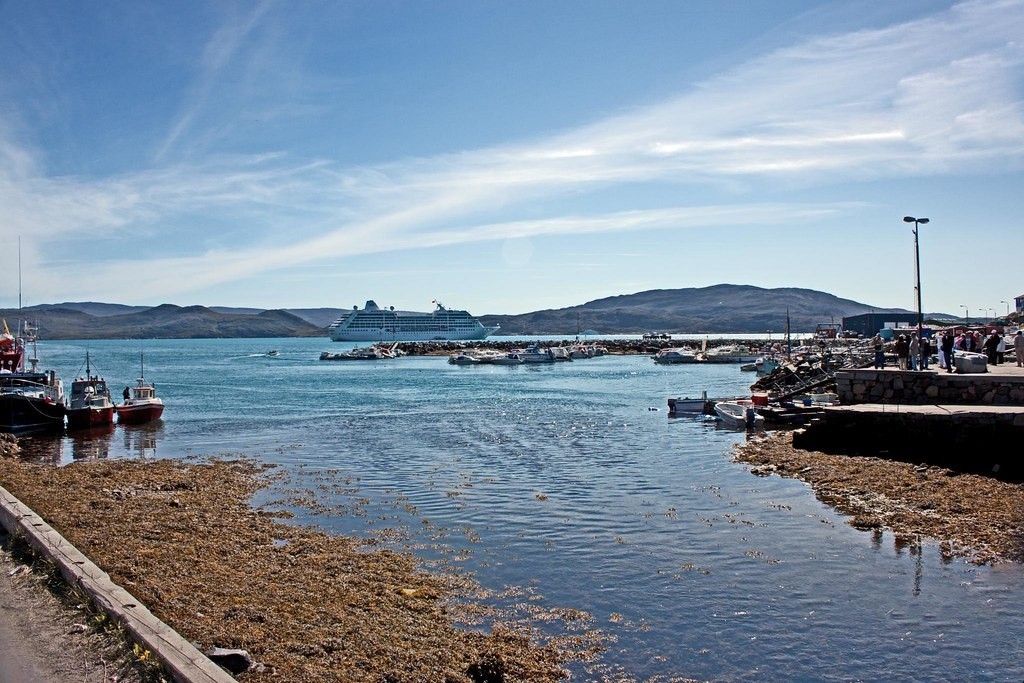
[329,300,501,342]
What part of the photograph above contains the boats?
[118,344,166,424]
[67,348,114,429]
[451,335,892,441]
[0,291,68,434]
[270,350,281,356]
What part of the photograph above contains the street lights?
[959,300,1009,325]
[319,343,408,362]
[902,217,931,370]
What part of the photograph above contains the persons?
[443,334,610,369]
[1,327,156,415]
[265,348,277,354]
[855,294,1024,383]
[651,323,855,385]
